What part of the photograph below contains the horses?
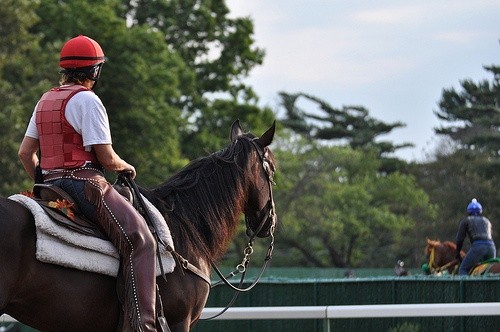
[0,119,278,332]
[423,236,500,276]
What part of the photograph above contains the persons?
[19,36,158,332]
[455,202,496,277]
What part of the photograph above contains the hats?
[59,35,106,68]
[467,202,483,213]
